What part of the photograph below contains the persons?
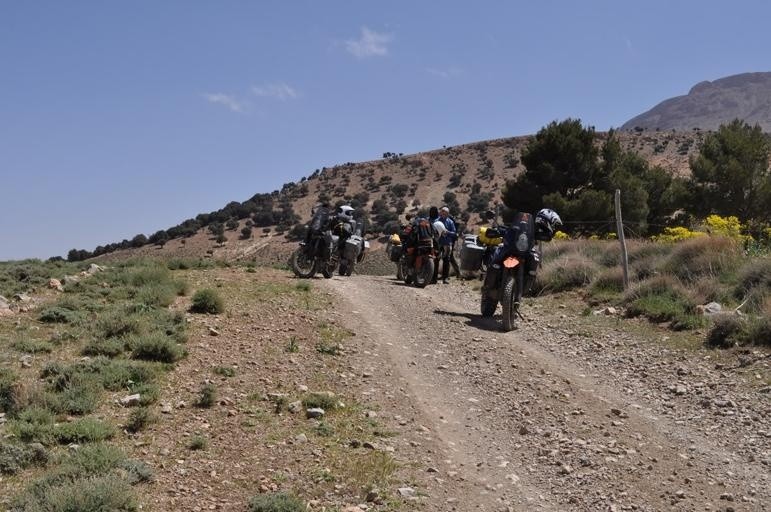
[428,207,464,284]
[329,205,355,266]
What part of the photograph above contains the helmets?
[536,208,563,234]
[432,221,447,239]
[337,205,355,220]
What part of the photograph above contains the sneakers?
[430,274,450,284]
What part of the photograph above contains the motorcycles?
[387,207,439,287]
[339,219,371,277]
[290,207,339,279]
[480,211,541,332]
